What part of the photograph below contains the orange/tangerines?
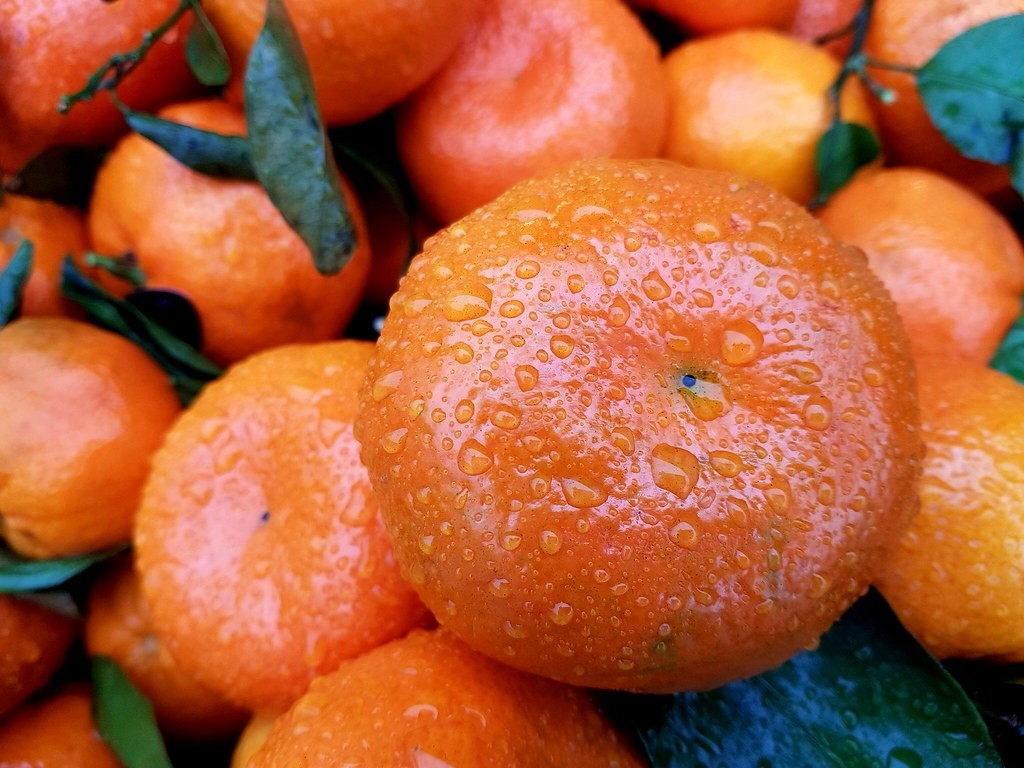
[0,0,1024,768]
